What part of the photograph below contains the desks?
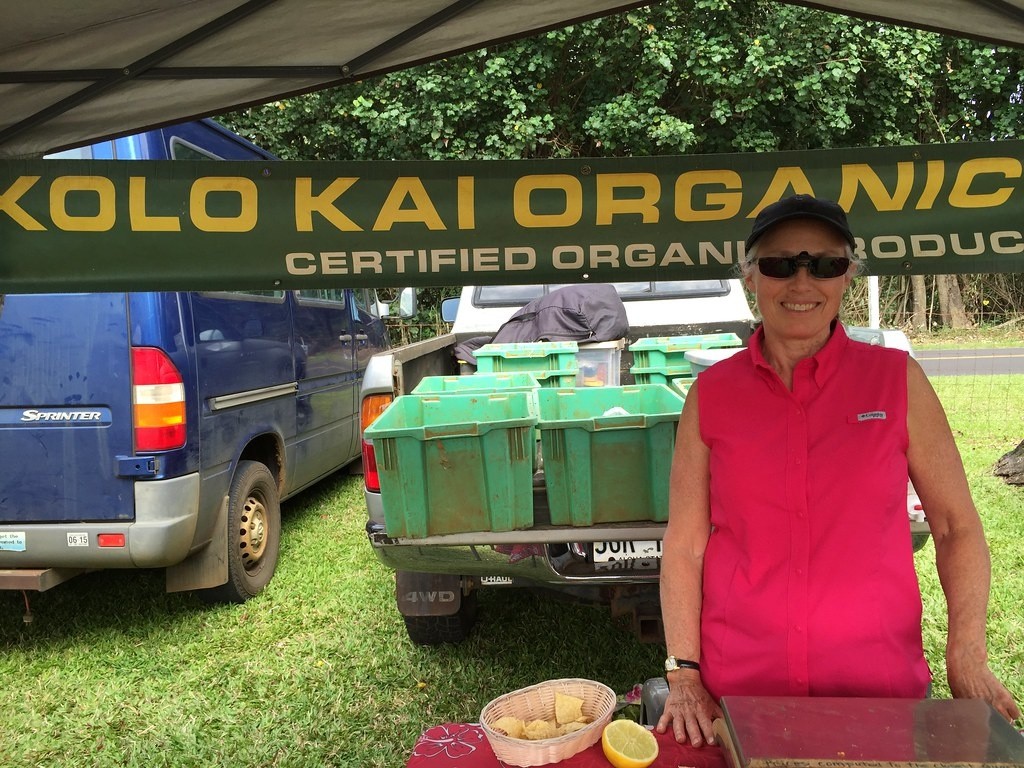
[407,723,733,768]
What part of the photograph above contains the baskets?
[480,677,617,767]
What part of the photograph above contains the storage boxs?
[361,333,750,539]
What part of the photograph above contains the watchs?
[665,656,700,672]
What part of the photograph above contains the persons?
[723,698,990,762]
[659,194,1020,746]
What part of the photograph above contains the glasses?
[747,253,854,281]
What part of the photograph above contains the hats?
[744,193,855,255]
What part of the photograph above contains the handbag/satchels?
[452,283,628,367]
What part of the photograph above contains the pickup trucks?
[359,284,932,646]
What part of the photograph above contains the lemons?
[602,719,659,768]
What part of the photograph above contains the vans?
[0,117,419,605]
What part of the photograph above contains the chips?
[488,690,597,742]
